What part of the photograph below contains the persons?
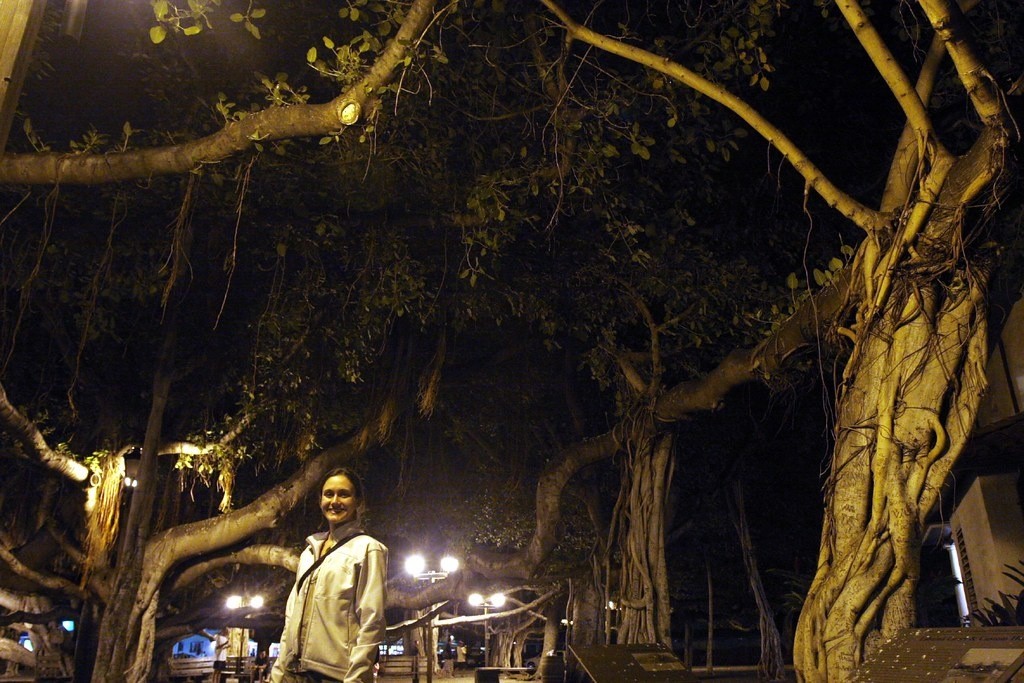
[265,468,388,683]
[255,650,270,683]
[212,627,231,683]
[438,639,467,679]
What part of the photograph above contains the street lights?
[405,552,460,681]
[467,591,506,667]
[226,594,265,659]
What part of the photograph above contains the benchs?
[379,655,436,683]
[475,667,533,683]
[220,656,278,683]
